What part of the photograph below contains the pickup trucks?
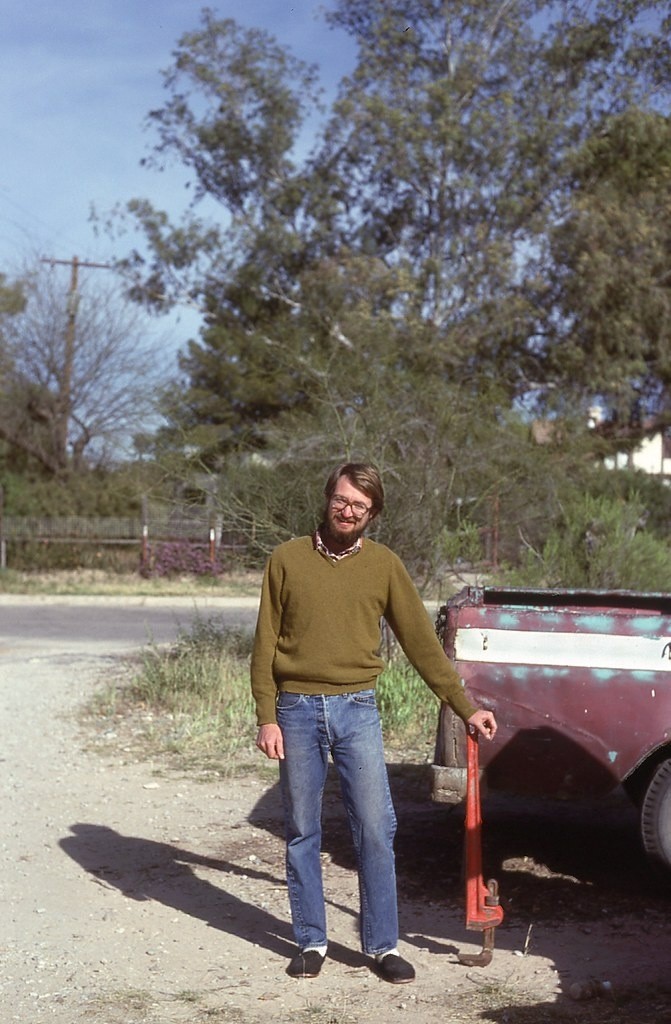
[429,582,671,897]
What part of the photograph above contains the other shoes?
[376,952,415,984]
[291,945,329,976]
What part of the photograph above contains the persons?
[249,463,498,984]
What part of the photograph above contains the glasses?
[329,494,370,518]
